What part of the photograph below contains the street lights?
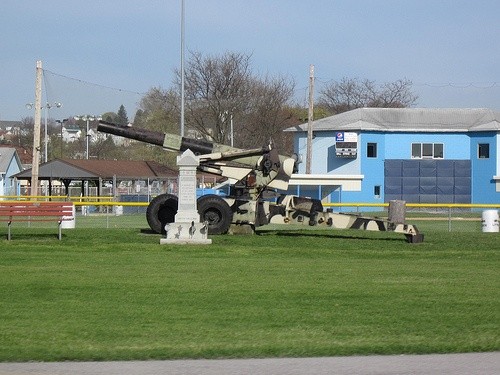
[73,115,104,159]
[25,101,62,163]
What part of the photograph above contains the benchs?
[0,202,75,241]
